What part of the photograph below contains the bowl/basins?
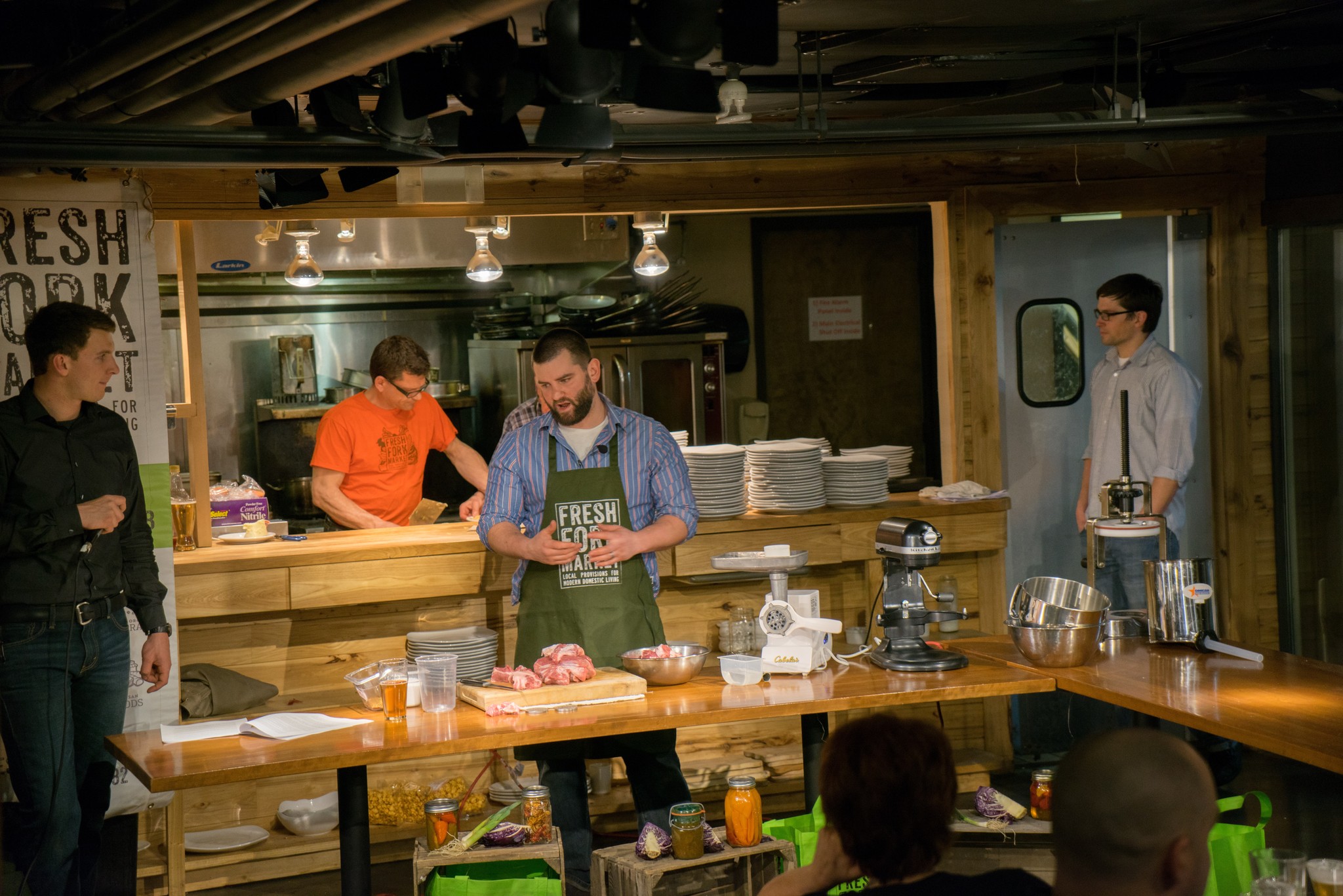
[276,791,340,838]
[1004,619,1104,669]
[1008,577,1111,629]
[615,645,710,686]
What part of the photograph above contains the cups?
[171,496,197,552]
[393,664,430,708]
[378,658,408,720]
[344,662,392,712]
[589,763,612,796]
[1305,858,1343,896]
[414,654,458,713]
[1249,848,1309,896]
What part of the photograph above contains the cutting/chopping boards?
[455,666,648,712]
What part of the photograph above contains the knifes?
[460,678,515,690]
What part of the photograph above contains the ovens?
[469,329,729,448]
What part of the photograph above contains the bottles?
[520,785,553,845]
[667,803,705,860]
[424,798,458,853]
[170,465,190,547]
[1030,770,1055,822]
[724,775,762,848]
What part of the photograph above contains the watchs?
[147,622,173,638]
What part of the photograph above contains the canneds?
[405,765,1053,866]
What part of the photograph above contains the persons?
[1073,273,1205,635]
[457,364,613,521]
[752,708,1053,896]
[1048,727,1217,896]
[0,301,175,896]
[475,327,699,896]
[311,334,490,533]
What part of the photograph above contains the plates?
[217,532,276,544]
[669,431,913,522]
[185,824,270,855]
[405,625,499,682]
[487,773,593,808]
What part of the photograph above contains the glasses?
[1093,308,1140,321]
[388,376,430,399]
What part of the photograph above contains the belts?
[0,586,128,627]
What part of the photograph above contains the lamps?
[464,217,513,285]
[629,211,670,279]
[261,220,283,244]
[333,219,358,245]
[283,220,325,288]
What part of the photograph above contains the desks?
[109,639,1053,887]
[945,611,1343,896]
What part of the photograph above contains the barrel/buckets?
[728,605,757,657]
[1142,558,1221,644]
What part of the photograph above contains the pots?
[266,476,327,520]
[556,268,712,336]
